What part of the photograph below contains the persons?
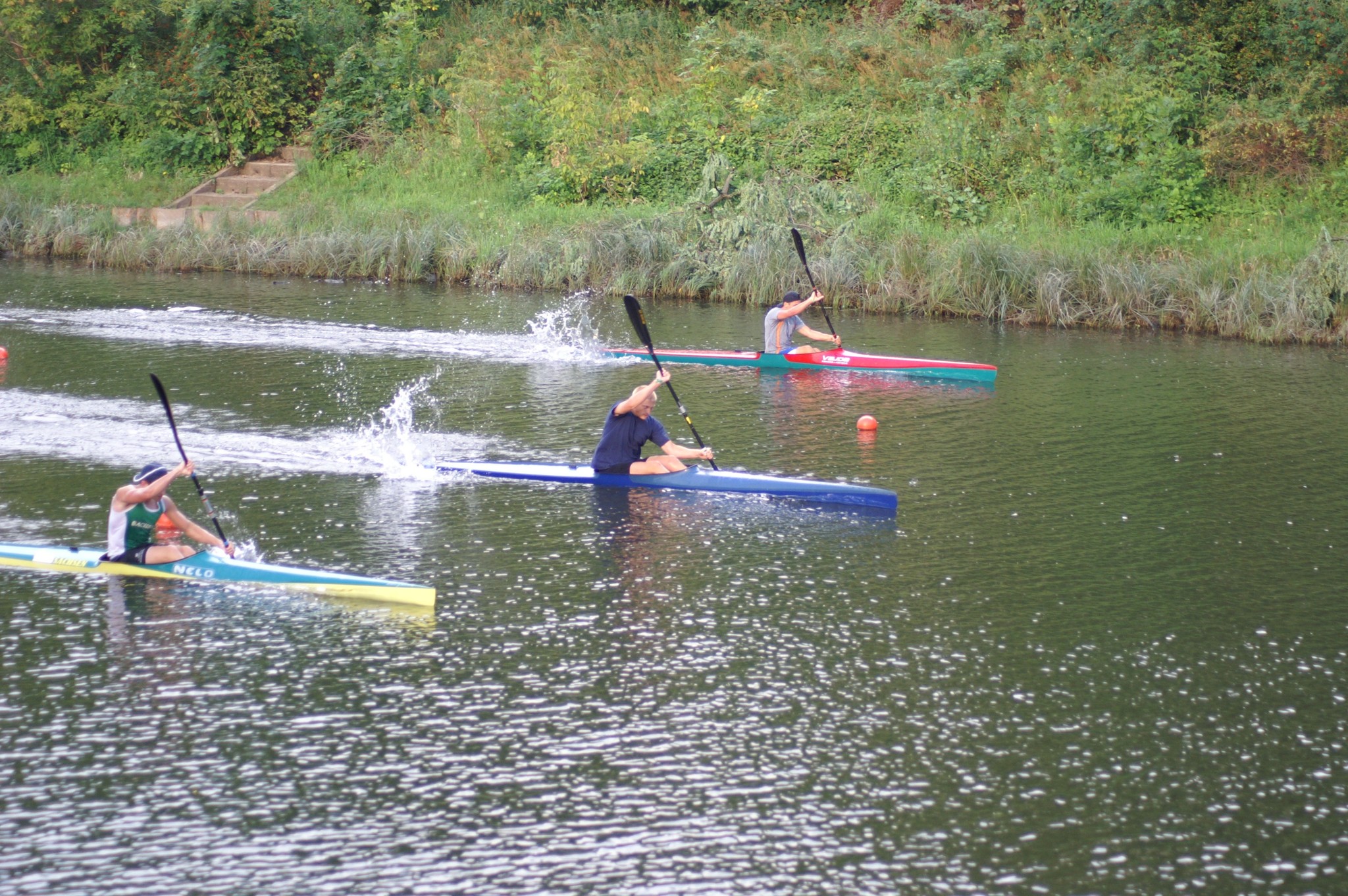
[108,459,235,565]
[765,290,841,354]
[591,368,713,475]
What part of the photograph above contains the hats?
[133,462,167,485]
[776,292,801,308]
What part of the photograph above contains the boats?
[434,463,897,510]
[0,541,436,606]
[604,347,998,382]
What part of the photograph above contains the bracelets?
[654,378,664,385]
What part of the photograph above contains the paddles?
[789,227,842,352]
[623,291,721,475]
[149,372,237,565]
